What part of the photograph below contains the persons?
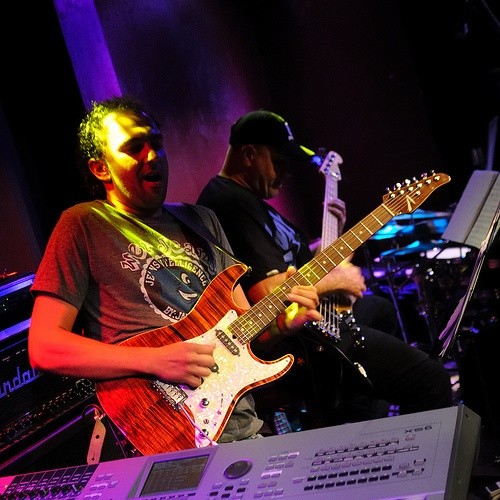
[27,95,450,461]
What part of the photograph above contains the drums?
[420,246,475,287]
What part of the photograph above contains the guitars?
[300,150,376,383]
[94,170,452,456]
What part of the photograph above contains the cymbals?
[366,225,429,261]
[390,211,451,221]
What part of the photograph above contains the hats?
[228,110,316,158]
